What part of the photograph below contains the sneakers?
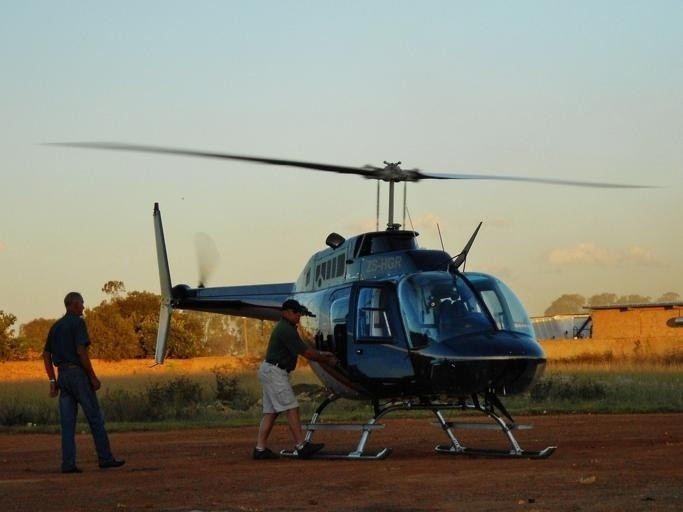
[252,447,281,459]
[297,442,323,459]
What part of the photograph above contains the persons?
[42,292,125,474]
[253,299,336,460]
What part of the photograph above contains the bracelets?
[48,379,57,383]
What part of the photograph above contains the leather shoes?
[63,467,82,473]
[100,458,124,469]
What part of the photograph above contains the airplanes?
[577,299,682,330]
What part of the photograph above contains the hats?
[282,300,303,310]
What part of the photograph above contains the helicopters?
[44,142,657,459]
